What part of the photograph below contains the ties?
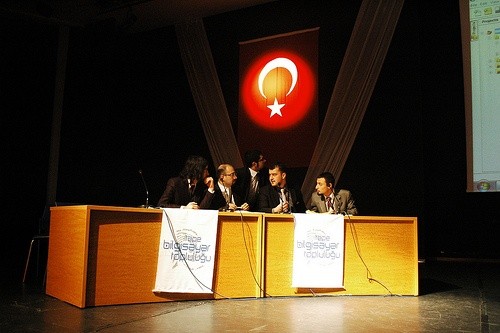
[190,185,195,196]
[250,175,258,194]
[327,197,331,210]
[280,189,286,205]
[223,189,229,204]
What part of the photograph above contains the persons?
[203,164,250,211]
[260,162,305,215]
[229,149,268,212]
[156,156,215,209]
[306,172,358,215]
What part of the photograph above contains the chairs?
[22,202,83,289]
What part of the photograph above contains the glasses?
[220,172,238,177]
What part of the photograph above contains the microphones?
[328,184,341,214]
[139,170,152,208]
[219,178,229,212]
[278,182,292,214]
[328,182,342,214]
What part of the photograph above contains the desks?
[45,205,419,309]
